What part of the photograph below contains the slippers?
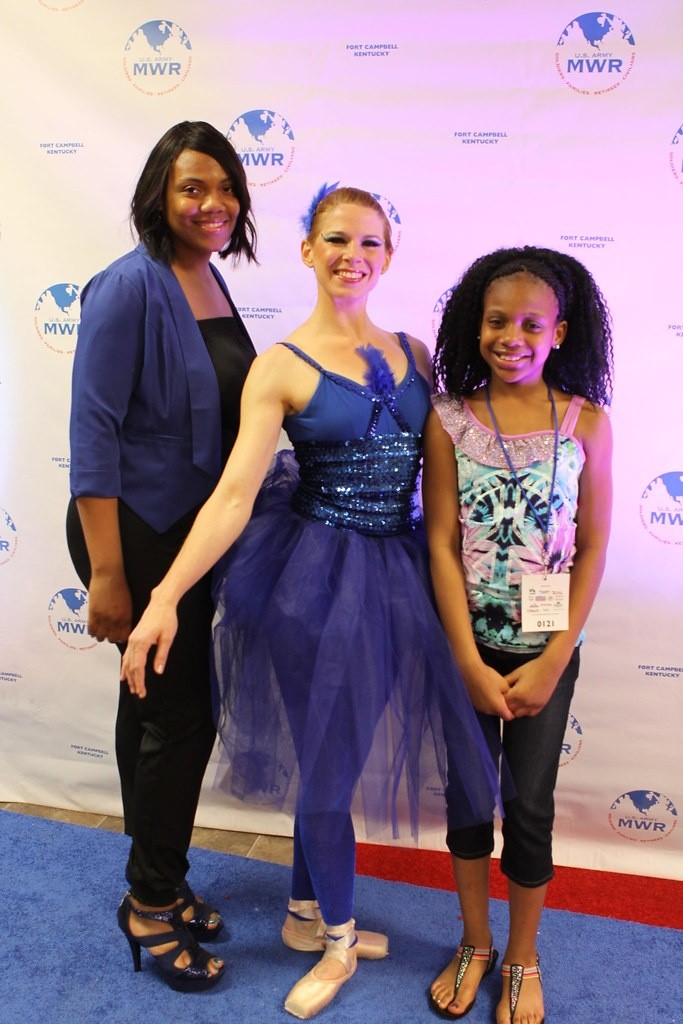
[492,951,545,1024]
[427,935,499,1019]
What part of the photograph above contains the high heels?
[117,891,224,993]
[173,881,222,942]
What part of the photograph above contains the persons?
[422,243,616,1023]
[118,186,439,1024]
[63,118,263,996]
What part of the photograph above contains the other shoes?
[284,948,357,1020]
[282,922,389,960]
